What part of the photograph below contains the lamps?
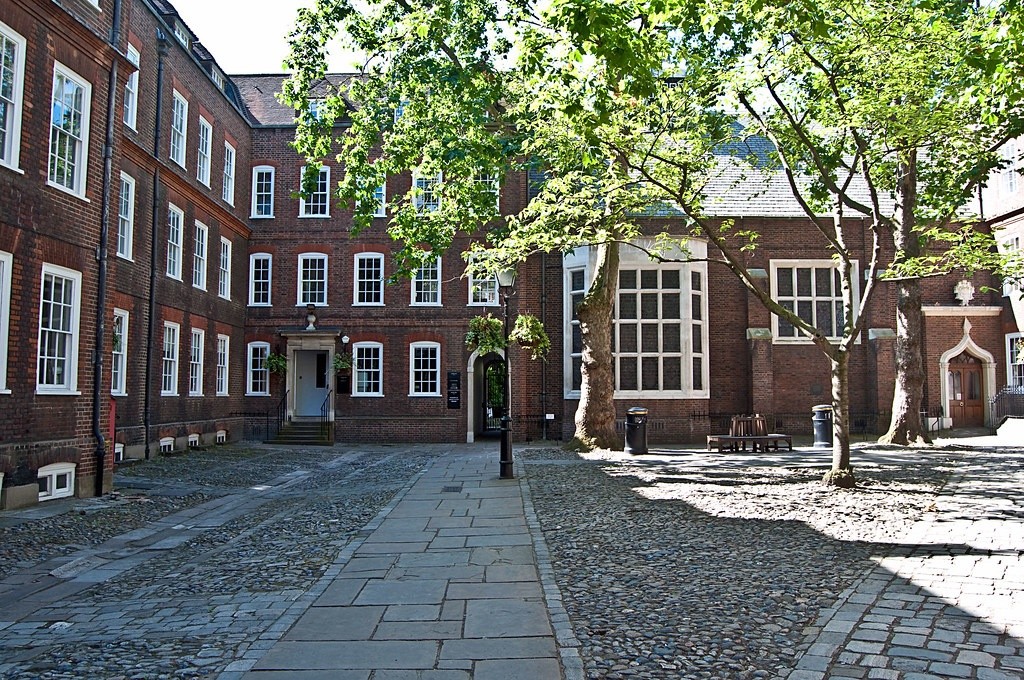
[305,305,318,331]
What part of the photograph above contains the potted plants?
[324,352,352,376]
[260,353,289,376]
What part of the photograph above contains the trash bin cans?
[812,404,833,448]
[624,407,649,454]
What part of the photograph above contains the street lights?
[496,260,518,480]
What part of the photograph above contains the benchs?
[706,414,792,453]
